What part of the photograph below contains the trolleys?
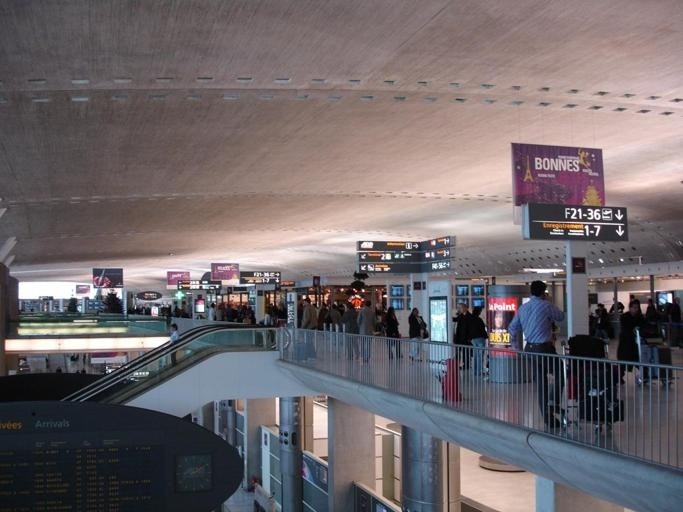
[558,331,623,438]
[633,326,677,389]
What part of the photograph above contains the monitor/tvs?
[522,295,530,304]
[589,293,598,304]
[656,291,674,306]
[471,285,484,295]
[472,299,484,307]
[456,298,469,308]
[455,285,468,297]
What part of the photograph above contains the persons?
[167,322,178,367]
[468,305,487,377]
[507,280,570,429]
[133,296,376,363]
[406,307,427,362]
[588,290,680,389]
[453,302,472,370]
[384,306,403,360]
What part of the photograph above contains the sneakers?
[546,417,561,429]
[547,404,568,415]
[347,352,424,369]
